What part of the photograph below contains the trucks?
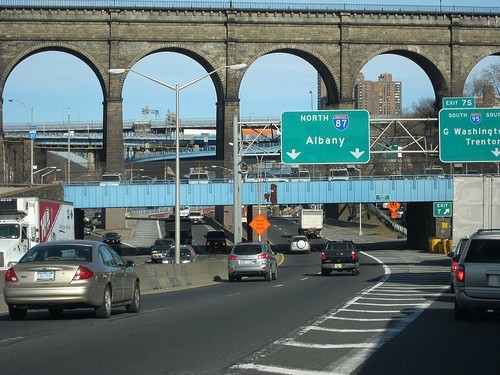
[295,209,324,238]
[0,196,75,272]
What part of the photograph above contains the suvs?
[327,168,351,181]
[100,172,123,187]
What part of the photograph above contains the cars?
[3,239,141,321]
[288,235,313,254]
[423,166,445,179]
[377,202,404,219]
[82,212,123,247]
[446,228,500,322]
[151,205,204,264]
[228,240,278,281]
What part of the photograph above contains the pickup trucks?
[320,240,362,276]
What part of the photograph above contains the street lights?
[106,63,248,266]
[30,140,57,185]
[8,98,34,123]
[41,168,62,185]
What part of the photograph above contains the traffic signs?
[438,107,500,163]
[280,109,372,166]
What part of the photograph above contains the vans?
[288,170,311,183]
[189,169,212,185]
[203,230,229,252]
